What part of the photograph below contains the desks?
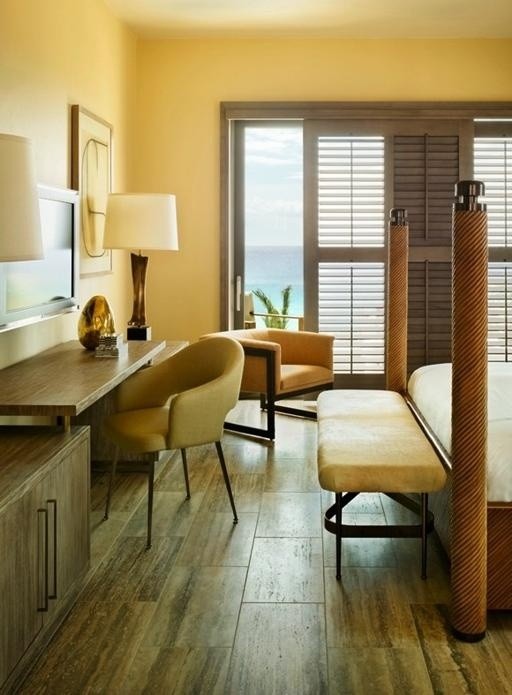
[0,341,166,427]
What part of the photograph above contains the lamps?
[0,134,44,263]
[103,192,179,341]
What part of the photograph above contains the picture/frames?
[70,104,114,278]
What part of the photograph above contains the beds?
[404,361,512,612]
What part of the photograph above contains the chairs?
[200,328,333,440]
[102,336,246,551]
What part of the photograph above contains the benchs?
[316,389,446,582]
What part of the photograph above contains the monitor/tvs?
[0,182,81,334]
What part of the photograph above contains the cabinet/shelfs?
[70,341,188,475]
[0,425,91,695]
